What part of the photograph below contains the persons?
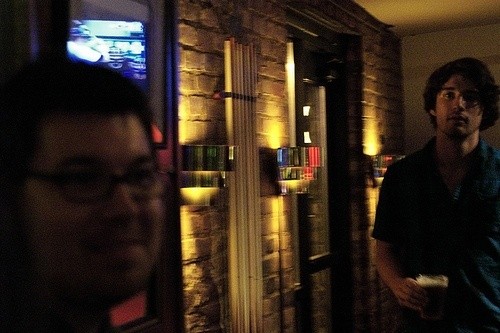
[0,61,165,333]
[371,57,500,333]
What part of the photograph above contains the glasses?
[26,166,176,202]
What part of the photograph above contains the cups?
[418,274,449,320]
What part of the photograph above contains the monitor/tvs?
[66,19,147,80]
